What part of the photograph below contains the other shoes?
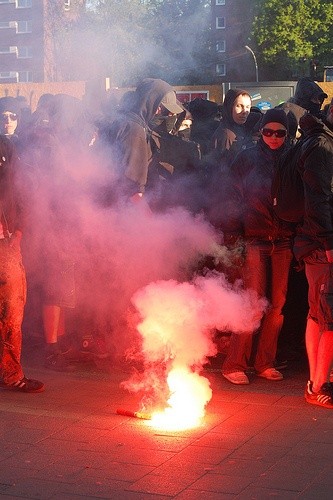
[79,333,115,358]
[45,344,72,359]
[23,337,43,347]
[275,359,288,370]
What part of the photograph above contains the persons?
[1,77,333,408]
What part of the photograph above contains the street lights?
[244,46,258,81]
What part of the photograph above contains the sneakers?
[304,380,333,408]
[0,374,44,392]
[253,367,283,380]
[221,370,249,384]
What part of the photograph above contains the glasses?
[261,128,287,138]
[312,97,323,102]
[0,113,18,121]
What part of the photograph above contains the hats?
[160,89,183,115]
